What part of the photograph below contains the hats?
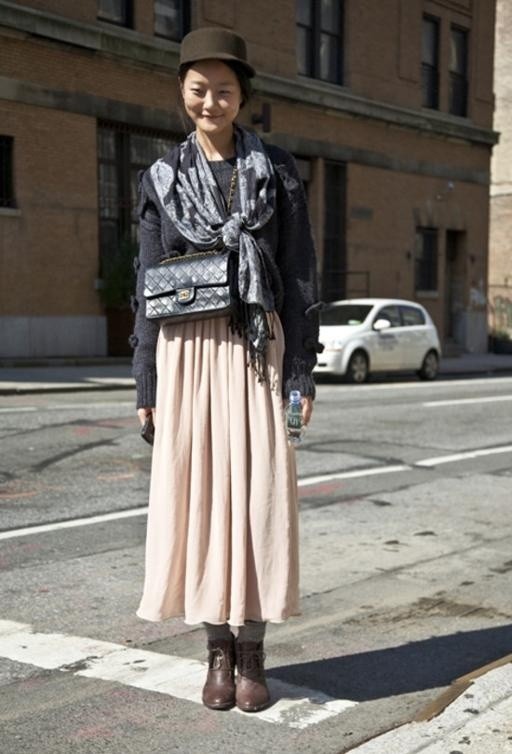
[176,24,255,79]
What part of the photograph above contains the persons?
[128,27,325,711]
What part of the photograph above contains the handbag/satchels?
[140,248,240,328]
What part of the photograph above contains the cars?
[306,299,445,385]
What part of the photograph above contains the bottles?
[284,392,306,446]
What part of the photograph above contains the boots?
[201,638,269,712]
